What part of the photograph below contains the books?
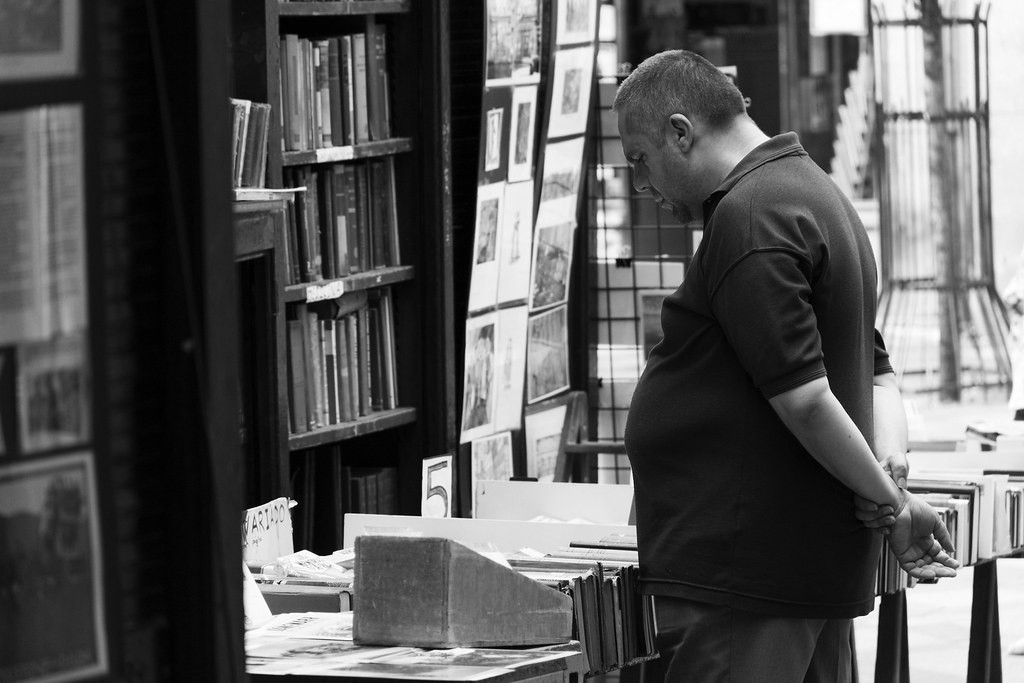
[230,0,658,683]
[873,469,1024,597]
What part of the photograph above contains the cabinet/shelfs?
[229,0,458,556]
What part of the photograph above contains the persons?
[610,49,960,683]
[25,373,98,673]
[463,326,492,432]
